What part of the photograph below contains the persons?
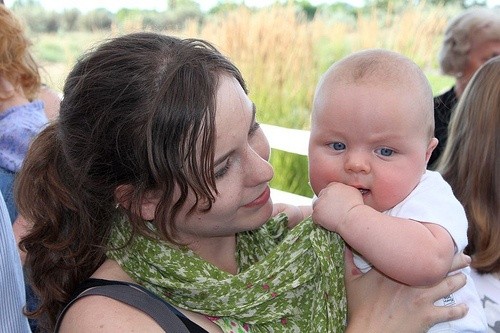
[270,50,490,333]
[428,6,500,166]
[13,33,472,333]
[0,1,62,333]
[433,56,500,333]
